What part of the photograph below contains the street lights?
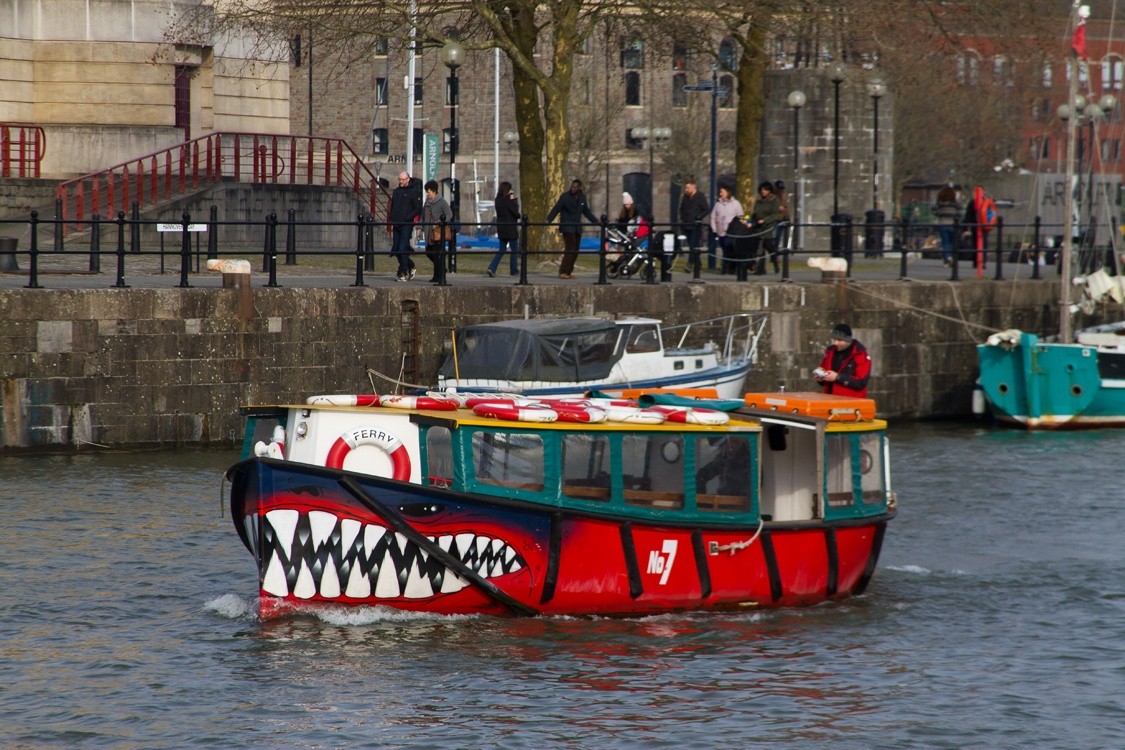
[439,41,467,275]
[786,89,808,251]
[827,59,850,212]
[865,78,887,212]
[1056,95,1116,274]
[630,126,673,177]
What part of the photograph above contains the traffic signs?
[682,85,712,91]
[715,86,729,98]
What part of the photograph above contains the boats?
[218,384,900,622]
[366,308,771,398]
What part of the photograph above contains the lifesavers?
[272,425,285,455]
[305,394,460,411]
[325,426,411,483]
[465,397,730,426]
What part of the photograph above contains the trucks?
[1036,174,1125,272]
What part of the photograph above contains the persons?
[680,181,709,273]
[416,180,452,281]
[710,179,790,273]
[815,324,871,398]
[544,178,598,278]
[931,186,1058,268]
[696,437,753,498]
[487,182,520,277]
[389,171,422,282]
[616,192,639,232]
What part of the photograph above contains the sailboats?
[973,1,1125,433]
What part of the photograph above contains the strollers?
[603,227,681,281]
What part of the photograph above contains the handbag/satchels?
[431,218,452,242]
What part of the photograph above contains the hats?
[622,192,634,205]
[831,323,852,342]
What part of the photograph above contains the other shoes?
[486,269,495,277]
[945,257,953,267]
[754,269,766,276]
[395,276,408,282]
[410,266,416,280]
[685,265,691,272]
[430,277,439,281]
[511,270,519,275]
[773,261,779,273]
[559,273,576,278]
